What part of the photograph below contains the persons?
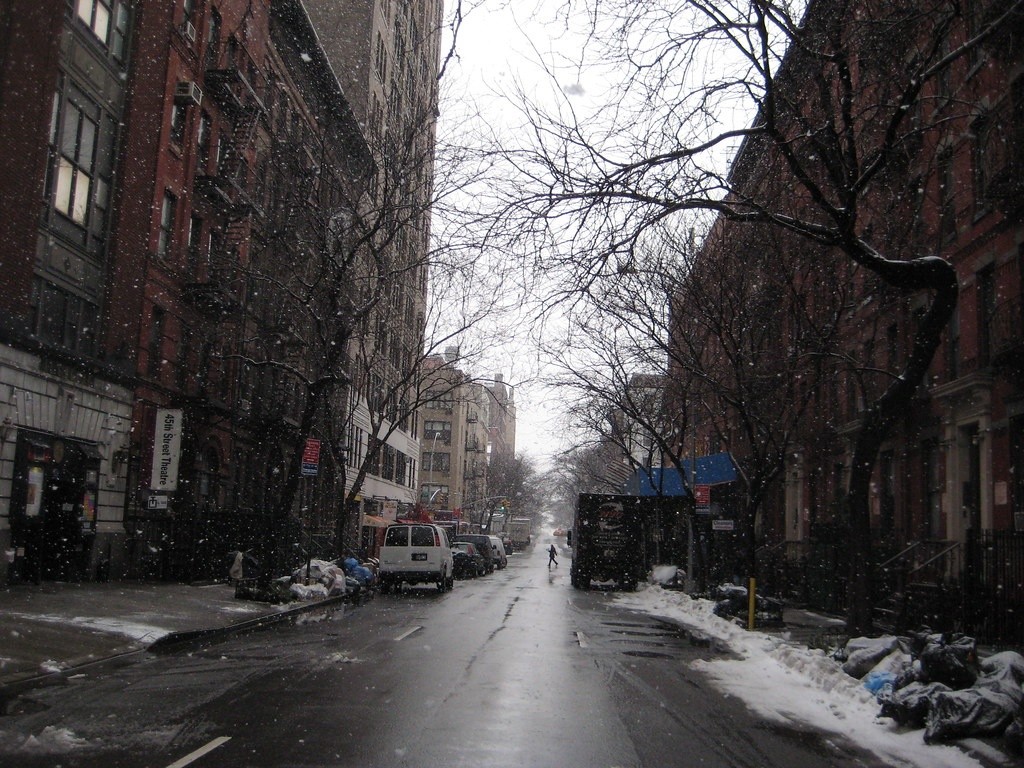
[546,545,558,566]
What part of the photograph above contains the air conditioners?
[28,445,45,461]
[173,81,203,107]
[179,21,196,43]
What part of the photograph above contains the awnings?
[362,514,398,527]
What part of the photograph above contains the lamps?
[373,494,413,506]
[971,436,985,445]
[105,412,122,425]
[12,387,32,402]
[0,416,14,448]
[939,443,952,452]
[111,444,129,474]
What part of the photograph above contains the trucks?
[566,492,647,592]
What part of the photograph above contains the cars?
[448,541,487,581]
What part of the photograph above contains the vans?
[378,522,454,593]
[449,517,531,572]
